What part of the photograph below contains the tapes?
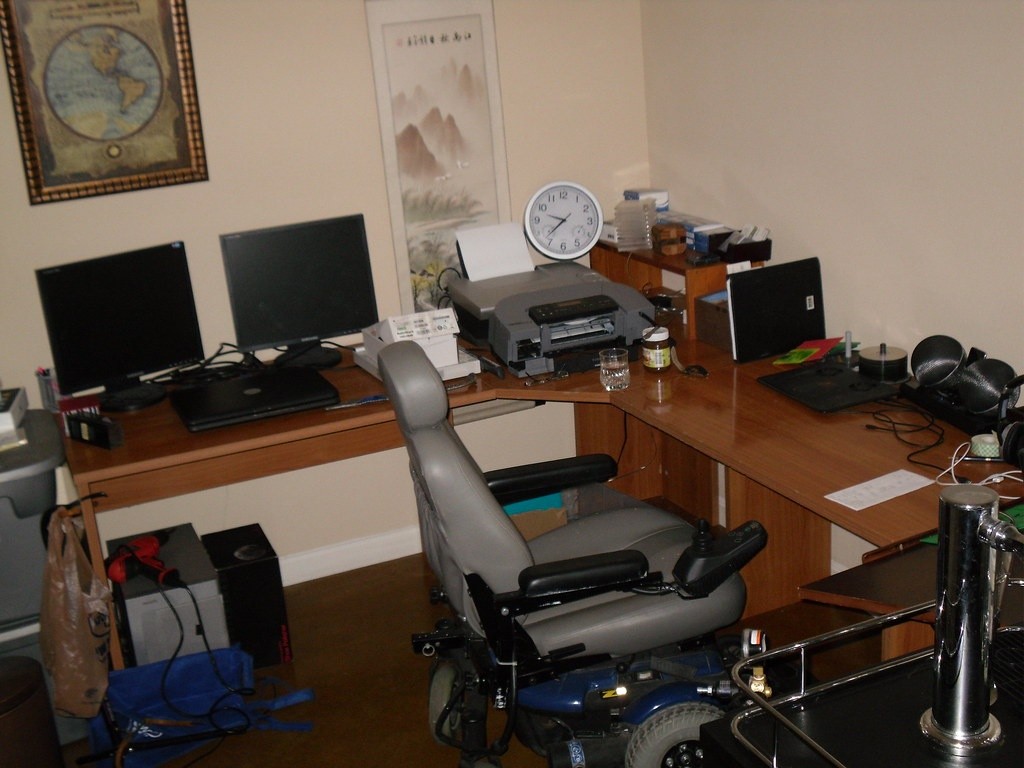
[970,433,999,457]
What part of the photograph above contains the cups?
[599,349,631,391]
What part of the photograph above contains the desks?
[58,286,1024,681]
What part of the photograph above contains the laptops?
[169,360,342,432]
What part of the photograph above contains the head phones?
[998,374,1024,471]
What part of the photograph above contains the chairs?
[376,339,769,767]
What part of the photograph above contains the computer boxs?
[202,523,297,670]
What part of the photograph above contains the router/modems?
[758,330,900,413]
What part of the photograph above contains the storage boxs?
[0,407,65,620]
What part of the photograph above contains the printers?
[449,239,657,379]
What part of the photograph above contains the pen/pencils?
[962,457,1004,462]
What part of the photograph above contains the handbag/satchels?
[87,646,253,768]
[39,509,113,718]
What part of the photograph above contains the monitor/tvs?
[35,240,205,414]
[220,214,379,370]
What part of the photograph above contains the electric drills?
[105,523,189,592]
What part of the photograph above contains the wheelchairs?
[380,339,769,768]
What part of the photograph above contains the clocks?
[523,181,603,260]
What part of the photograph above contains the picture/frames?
[0,0,209,205]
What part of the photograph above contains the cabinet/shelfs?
[588,220,765,340]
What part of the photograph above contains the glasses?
[524,363,569,387]
